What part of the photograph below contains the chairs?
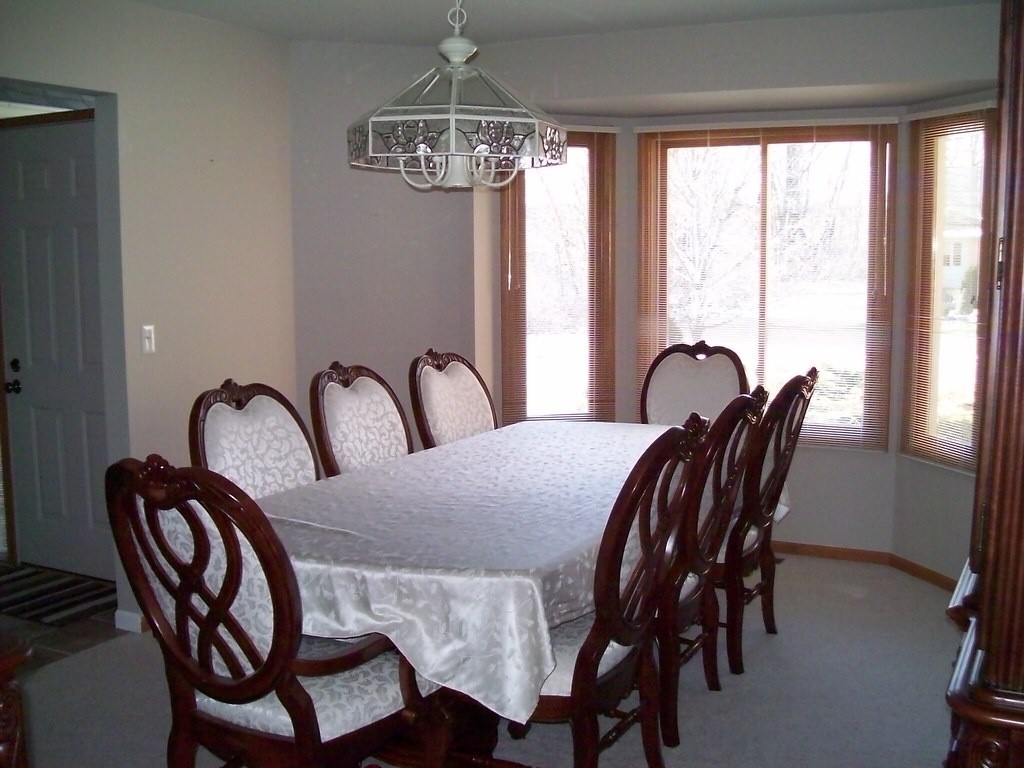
[102,340,818,767]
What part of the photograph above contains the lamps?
[347,0,568,191]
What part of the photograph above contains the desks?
[152,414,792,768]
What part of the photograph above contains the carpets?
[1,561,117,629]
